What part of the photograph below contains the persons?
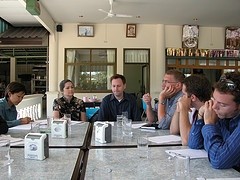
[144,70,186,130]
[0,82,31,127]
[53,80,86,121]
[170,75,212,146]
[188,72,240,172]
[99,74,142,121]
[0,115,8,134]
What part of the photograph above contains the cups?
[93,94,97,101]
[173,155,190,180]
[117,115,124,128]
[122,119,132,136]
[83,96,87,102]
[0,139,10,165]
[31,122,40,133]
[137,136,149,159]
[47,115,54,127]
[122,112,128,126]
[64,114,71,126]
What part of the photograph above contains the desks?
[0,119,240,180]
[83,101,101,118]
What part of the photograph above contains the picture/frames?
[126,24,136,37]
[78,25,94,37]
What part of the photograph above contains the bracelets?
[199,113,204,118]
[160,100,165,105]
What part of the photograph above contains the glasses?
[220,74,240,93]
[162,79,177,84]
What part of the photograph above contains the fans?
[98,0,133,20]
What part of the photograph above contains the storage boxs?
[96,123,111,143]
[52,121,68,138]
[24,133,49,160]
